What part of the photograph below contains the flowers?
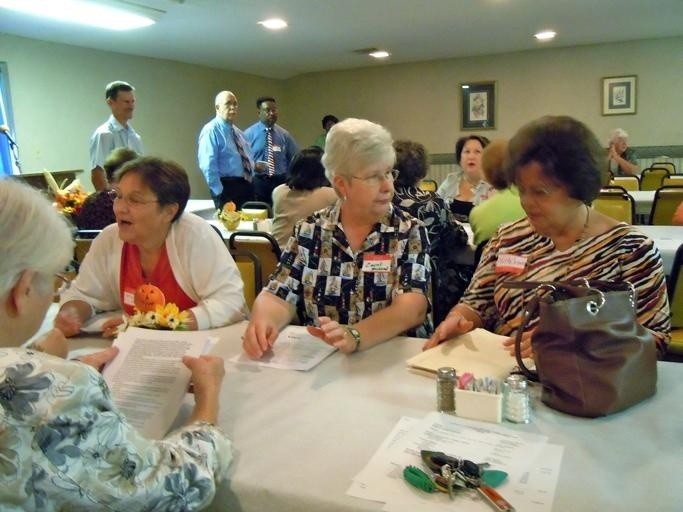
[45,178,91,217]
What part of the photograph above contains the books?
[91,326,218,444]
[401,323,532,392]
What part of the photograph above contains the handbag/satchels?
[502,277,657,417]
[430,221,476,323]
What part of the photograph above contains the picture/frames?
[601,74,638,115]
[457,79,498,132]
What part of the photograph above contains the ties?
[264,128,275,177]
[231,128,252,183]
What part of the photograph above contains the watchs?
[343,325,359,354]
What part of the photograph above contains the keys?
[401,450,515,512]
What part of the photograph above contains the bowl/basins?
[222,217,239,230]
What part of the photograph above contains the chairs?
[582,161,682,365]
[50,199,288,316]
[420,178,438,193]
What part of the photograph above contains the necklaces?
[526,204,590,255]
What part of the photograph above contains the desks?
[0,302,682,512]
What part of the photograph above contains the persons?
[244,95,300,200]
[238,117,429,360]
[51,155,246,340]
[196,92,254,215]
[86,82,144,193]
[73,113,649,300]
[422,112,671,364]
[0,174,232,511]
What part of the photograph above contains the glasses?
[111,186,159,207]
[350,169,400,186]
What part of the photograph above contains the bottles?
[252,219,257,230]
[437,367,457,413]
[503,376,529,423]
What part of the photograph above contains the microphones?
[0,125,19,149]
